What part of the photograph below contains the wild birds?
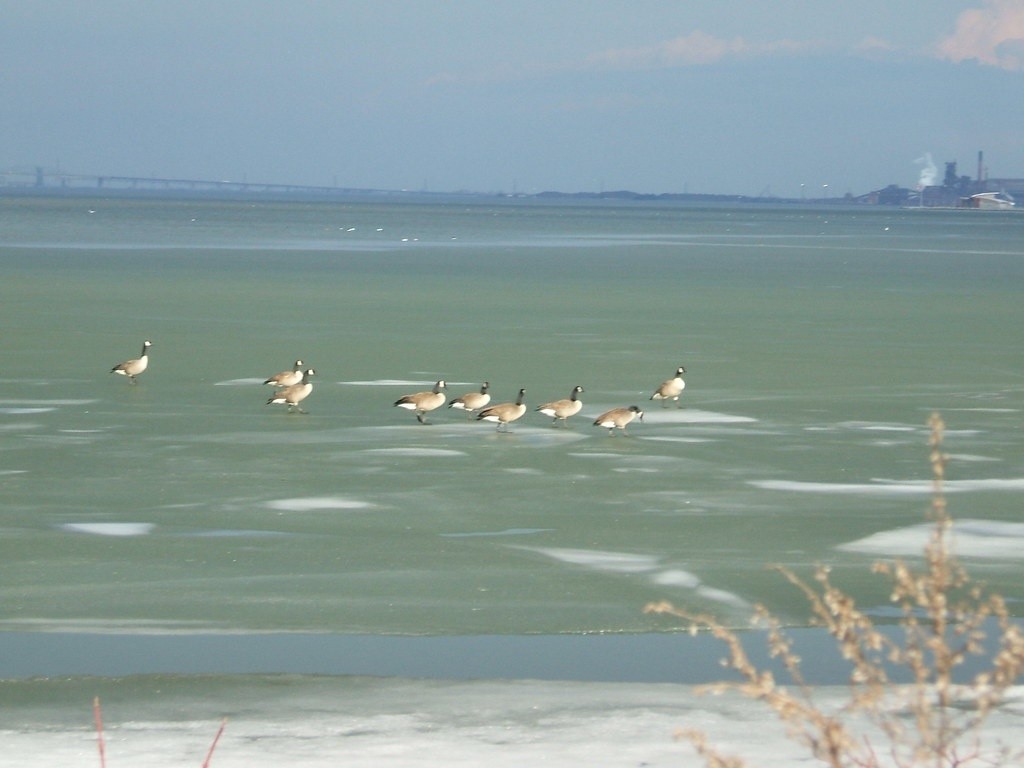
[262,359,317,415]
[448,381,492,421]
[592,404,644,438]
[650,366,690,409]
[534,385,585,429]
[108,340,156,385]
[477,387,527,434]
[392,380,448,425]
